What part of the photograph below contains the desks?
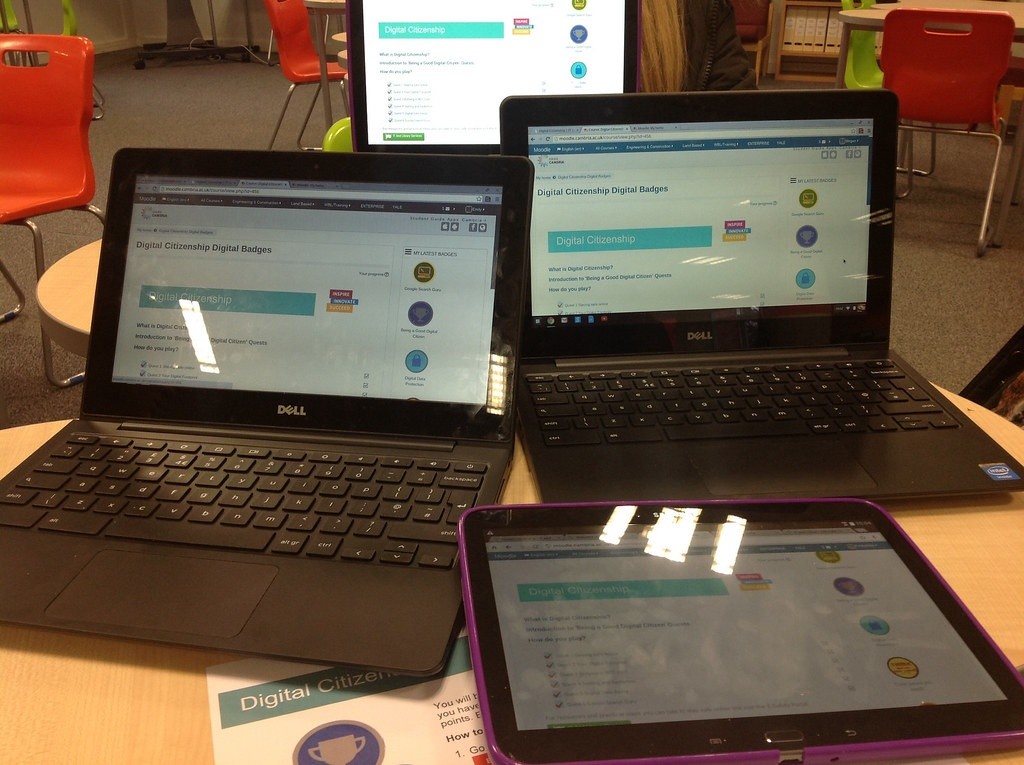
[838,0,1024,204]
[0,365,1024,765]
[35,236,102,358]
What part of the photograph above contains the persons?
[638,0,758,92]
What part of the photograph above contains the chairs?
[266,0,348,151]
[881,9,1014,257]
[840,0,937,199]
[0,34,104,387]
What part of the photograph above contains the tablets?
[458,498,1024,765]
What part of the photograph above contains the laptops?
[0,0,1024,674]
